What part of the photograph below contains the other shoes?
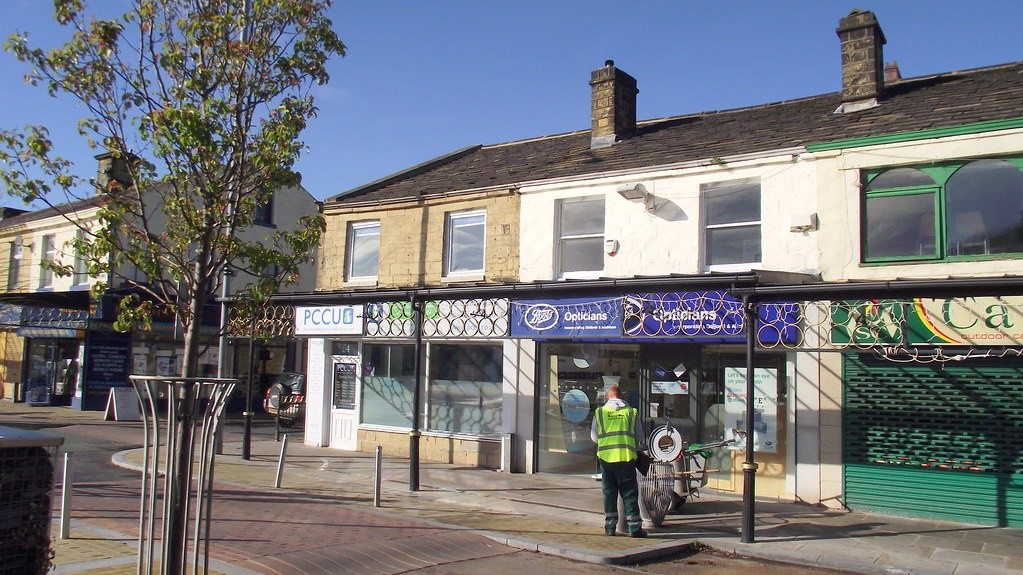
[605,530,616,536]
[627,528,648,538]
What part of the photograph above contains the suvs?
[262,373,307,428]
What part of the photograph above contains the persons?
[590,384,653,539]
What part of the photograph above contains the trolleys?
[642,448,714,513]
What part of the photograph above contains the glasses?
[732,428,747,439]
